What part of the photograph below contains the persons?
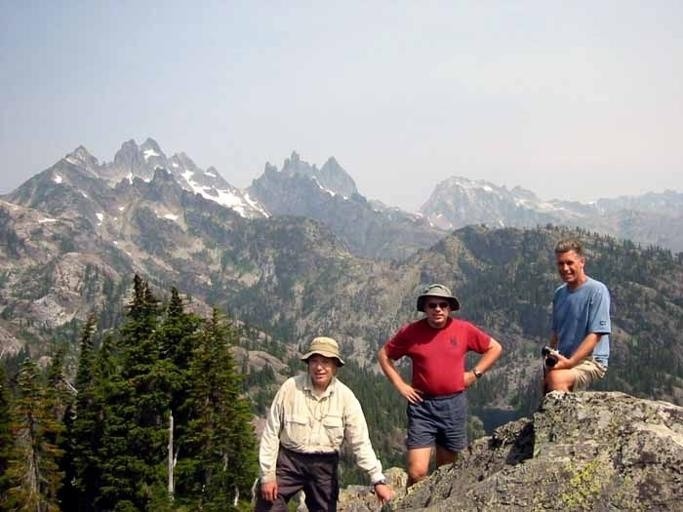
[375,282,503,487]
[540,239,612,395]
[254,335,397,512]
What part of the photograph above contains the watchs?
[472,368,482,378]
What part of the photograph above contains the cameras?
[542,345,558,367]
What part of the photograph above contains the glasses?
[422,302,450,309]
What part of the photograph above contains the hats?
[298,336,347,367]
[415,284,460,311]
